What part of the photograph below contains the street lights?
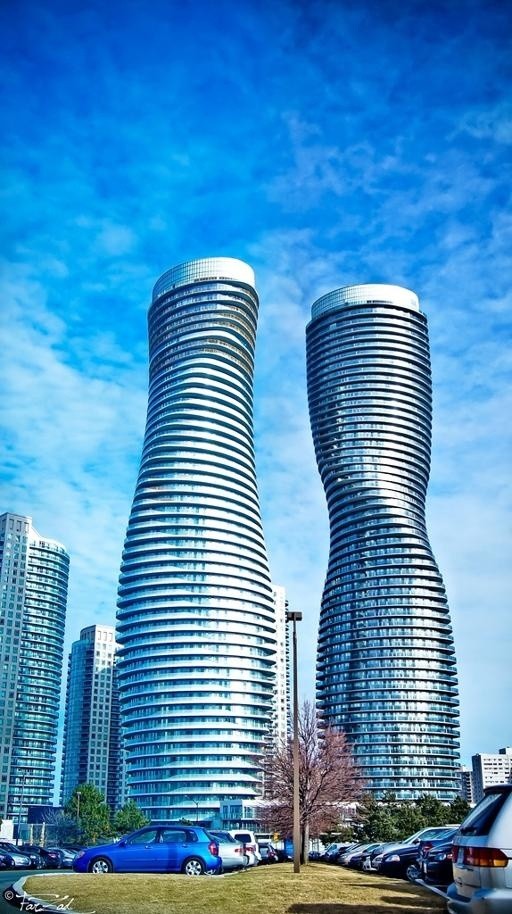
[77,791,81,816]
[185,794,198,822]
[287,613,302,874]
[16,774,25,846]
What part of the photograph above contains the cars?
[309,784,512,914]
[0,825,278,875]
